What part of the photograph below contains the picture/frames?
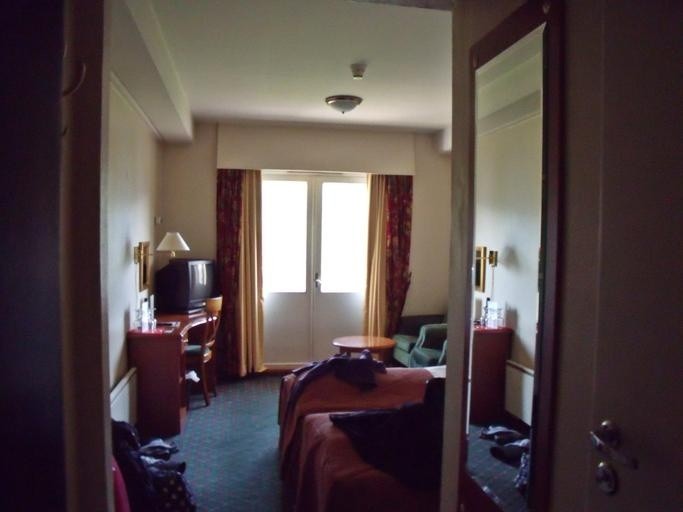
[475,247,485,293]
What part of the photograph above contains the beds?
[305,410,440,511]
[283,322,513,425]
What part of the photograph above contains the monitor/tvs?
[156,257,217,315]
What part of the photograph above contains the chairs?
[394,311,448,368]
[188,296,226,407]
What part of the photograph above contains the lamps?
[133,231,192,265]
[325,96,364,115]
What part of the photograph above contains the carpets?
[465,425,526,511]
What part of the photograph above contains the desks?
[125,311,220,438]
